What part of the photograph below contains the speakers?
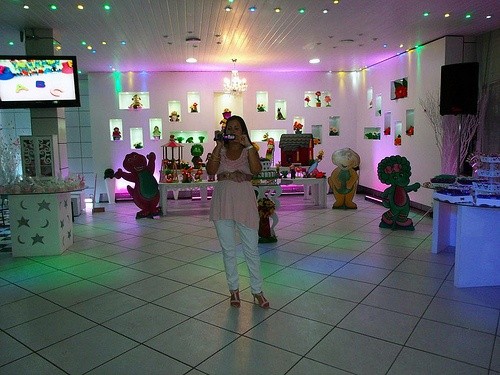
[439,62,479,116]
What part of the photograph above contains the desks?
[159,177,327,216]
[432,198,500,287]
[0,186,89,257]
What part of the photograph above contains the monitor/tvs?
[0,55,81,108]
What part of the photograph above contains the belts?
[217,170,254,183]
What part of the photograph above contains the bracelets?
[246,145,253,151]
[212,153,220,159]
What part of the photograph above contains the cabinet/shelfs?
[20,134,59,181]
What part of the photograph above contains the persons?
[205,115,269,309]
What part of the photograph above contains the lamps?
[224,59,249,92]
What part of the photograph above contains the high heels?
[252,290,269,308]
[230,288,241,307]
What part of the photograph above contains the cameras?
[217,133,235,141]
